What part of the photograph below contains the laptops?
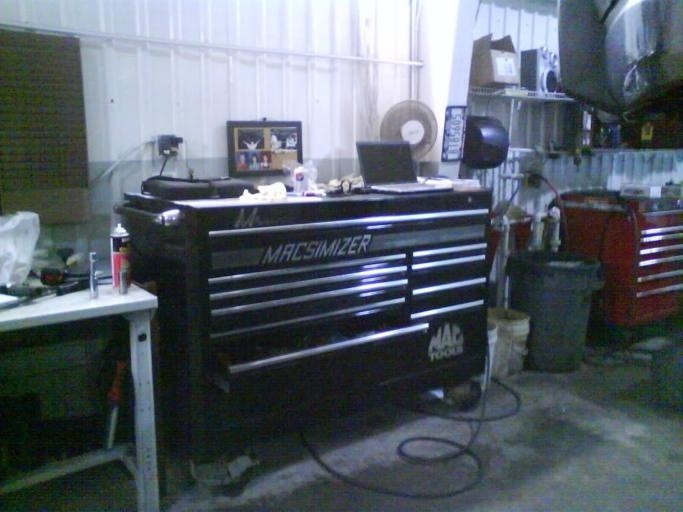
[356,141,453,195]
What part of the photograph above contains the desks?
[1,265,159,512]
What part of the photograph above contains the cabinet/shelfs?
[496,190,683,350]
[114,187,494,496]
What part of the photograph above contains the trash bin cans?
[506,246,600,368]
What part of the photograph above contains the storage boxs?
[473,34,520,87]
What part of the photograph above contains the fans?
[380,100,438,179]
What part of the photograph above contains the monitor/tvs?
[0,25,91,227]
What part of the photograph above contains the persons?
[238,154,248,172]
[286,133,297,149]
[243,139,261,150]
[250,155,262,171]
[262,154,270,171]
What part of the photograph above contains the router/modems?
[171,137,183,144]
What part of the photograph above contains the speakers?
[521,47,561,96]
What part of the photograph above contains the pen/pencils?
[287,191,326,197]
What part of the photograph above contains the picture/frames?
[226,117,303,178]
